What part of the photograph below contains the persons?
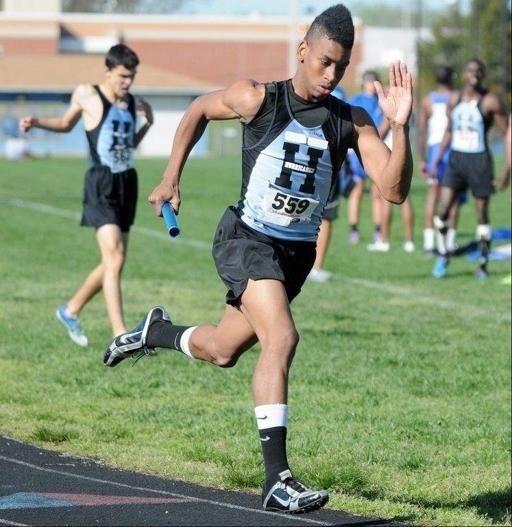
[17,44,154,347]
[417,59,512,285]
[101,3,415,514]
[329,71,415,253]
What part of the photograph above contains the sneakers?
[55,305,90,346]
[262,468,330,515]
[102,306,172,367]
[346,228,492,281]
